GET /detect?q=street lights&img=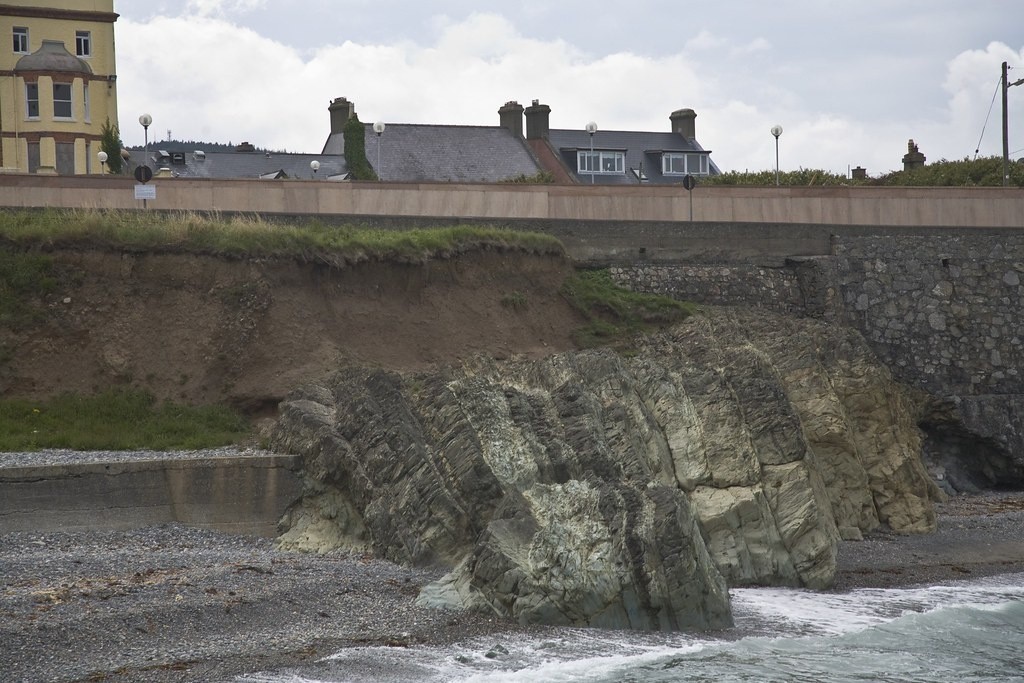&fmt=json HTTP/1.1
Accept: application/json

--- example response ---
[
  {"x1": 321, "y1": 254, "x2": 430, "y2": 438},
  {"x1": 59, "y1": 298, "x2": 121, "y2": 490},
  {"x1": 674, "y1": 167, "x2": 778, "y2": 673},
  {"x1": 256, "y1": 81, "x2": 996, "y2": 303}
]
[
  {"x1": 139, "y1": 113, "x2": 154, "y2": 167},
  {"x1": 770, "y1": 124, "x2": 784, "y2": 187},
  {"x1": 372, "y1": 120, "x2": 386, "y2": 183},
  {"x1": 309, "y1": 159, "x2": 320, "y2": 180},
  {"x1": 585, "y1": 121, "x2": 598, "y2": 186},
  {"x1": 97, "y1": 151, "x2": 109, "y2": 176}
]
